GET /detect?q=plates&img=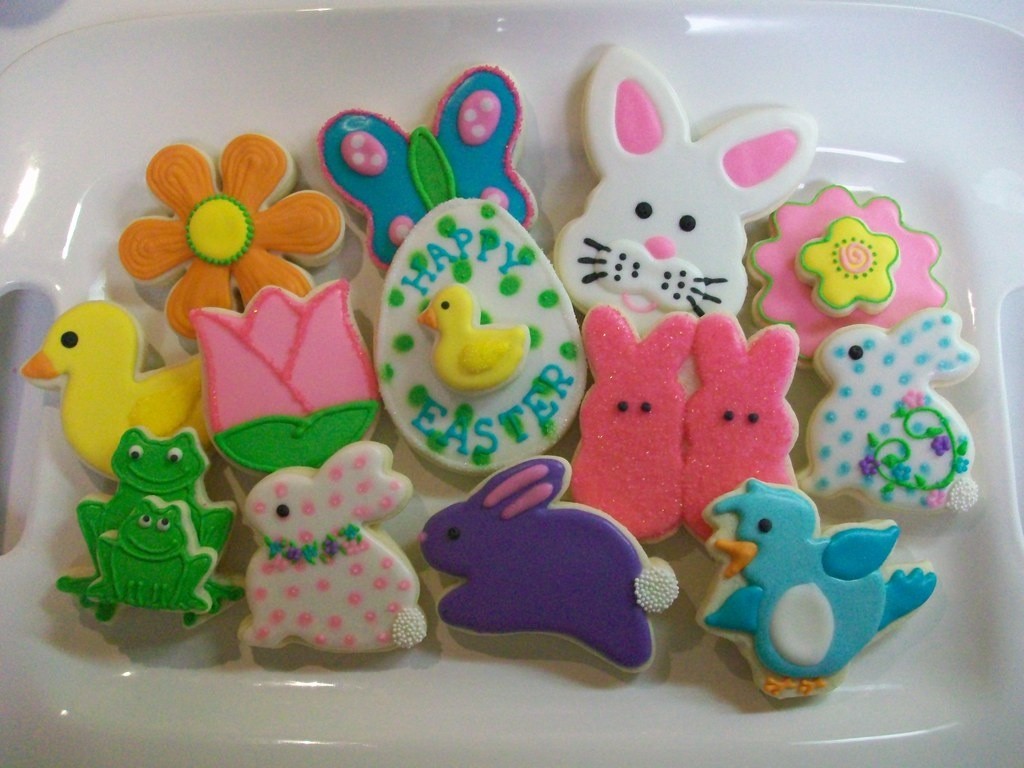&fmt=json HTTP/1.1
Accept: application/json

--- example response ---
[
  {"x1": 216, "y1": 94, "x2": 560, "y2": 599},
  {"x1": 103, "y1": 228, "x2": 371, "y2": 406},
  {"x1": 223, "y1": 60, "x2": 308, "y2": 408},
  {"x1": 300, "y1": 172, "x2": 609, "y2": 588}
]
[{"x1": 1, "y1": 2, "x2": 1024, "y2": 768}]
